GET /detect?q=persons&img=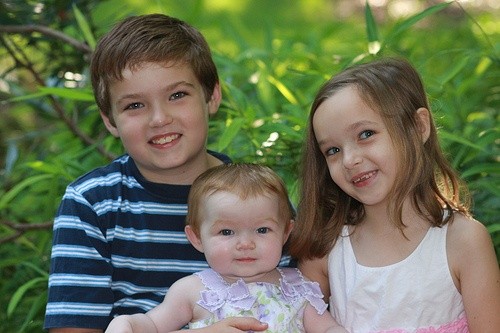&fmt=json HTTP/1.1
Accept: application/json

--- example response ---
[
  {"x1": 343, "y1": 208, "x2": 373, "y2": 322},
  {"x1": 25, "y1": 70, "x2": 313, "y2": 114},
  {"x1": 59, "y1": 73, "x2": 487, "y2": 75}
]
[
  {"x1": 287, "y1": 54, "x2": 500, "y2": 333},
  {"x1": 45, "y1": 12, "x2": 298, "y2": 333},
  {"x1": 105, "y1": 162, "x2": 351, "y2": 333}
]
